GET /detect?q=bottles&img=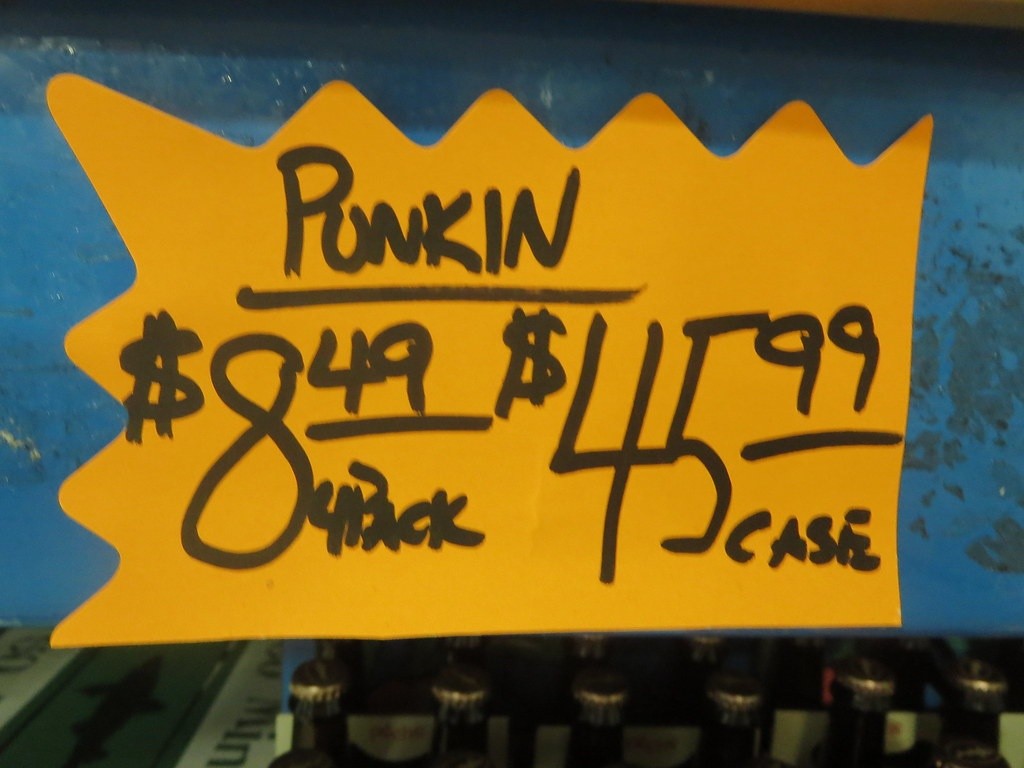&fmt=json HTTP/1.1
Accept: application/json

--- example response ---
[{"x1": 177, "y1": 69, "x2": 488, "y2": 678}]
[{"x1": 270, "y1": 637, "x2": 1022, "y2": 766}]
